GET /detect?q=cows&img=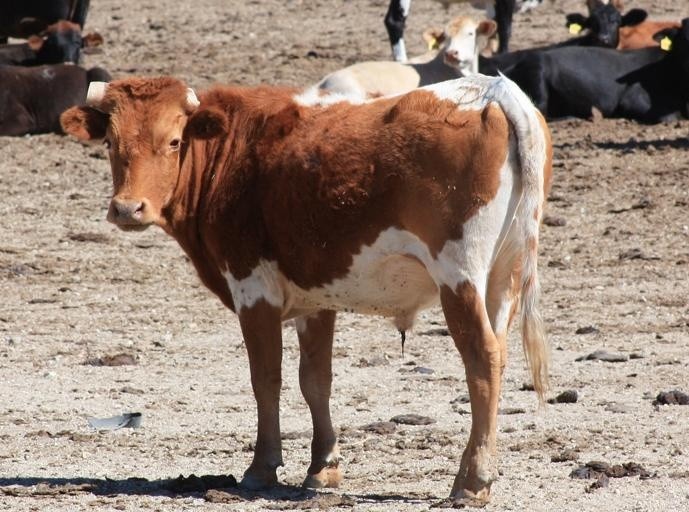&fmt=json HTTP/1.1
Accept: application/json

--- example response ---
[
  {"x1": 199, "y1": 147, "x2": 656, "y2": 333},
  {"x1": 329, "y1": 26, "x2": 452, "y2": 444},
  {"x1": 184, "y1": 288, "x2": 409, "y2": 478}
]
[
  {"x1": 479, "y1": 0, "x2": 649, "y2": 68},
  {"x1": 609, "y1": 0, "x2": 681, "y2": 49},
  {"x1": 1, "y1": 0, "x2": 115, "y2": 140},
  {"x1": 55, "y1": 66, "x2": 554, "y2": 510},
  {"x1": 505, "y1": 16, "x2": 689, "y2": 127},
  {"x1": 315, "y1": 17, "x2": 496, "y2": 103},
  {"x1": 382, "y1": 0, "x2": 544, "y2": 61}
]
